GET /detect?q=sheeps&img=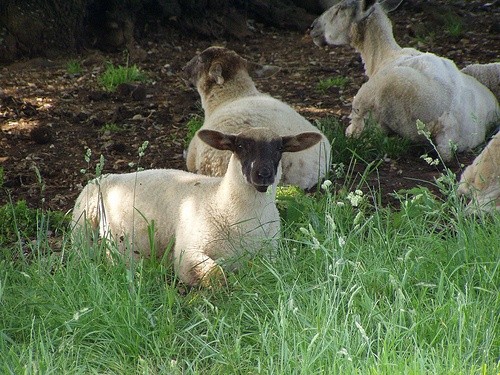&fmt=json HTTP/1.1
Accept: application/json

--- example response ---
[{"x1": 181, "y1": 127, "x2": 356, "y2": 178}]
[
  {"x1": 69, "y1": 126, "x2": 323, "y2": 289},
  {"x1": 455, "y1": 61, "x2": 500, "y2": 222},
  {"x1": 177, "y1": 44, "x2": 333, "y2": 193},
  {"x1": 309, "y1": 0, "x2": 500, "y2": 169}
]
